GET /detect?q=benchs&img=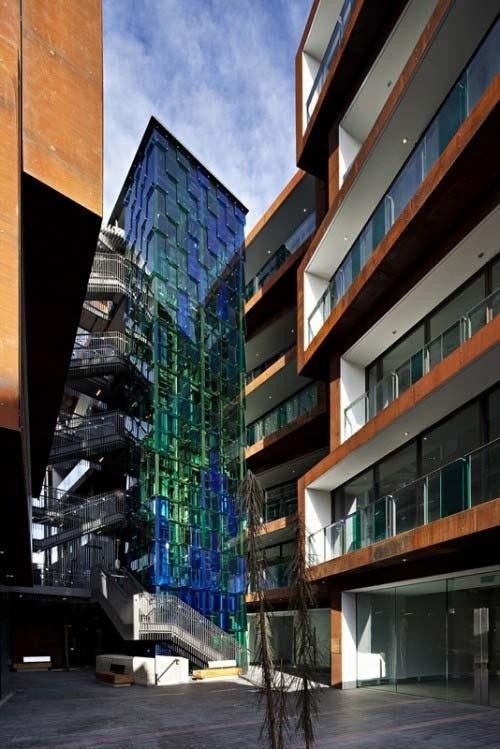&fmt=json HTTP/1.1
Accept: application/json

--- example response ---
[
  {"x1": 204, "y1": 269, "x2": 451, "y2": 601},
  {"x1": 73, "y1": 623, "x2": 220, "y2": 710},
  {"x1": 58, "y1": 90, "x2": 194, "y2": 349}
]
[
  {"x1": 192, "y1": 668, "x2": 242, "y2": 682},
  {"x1": 13, "y1": 662, "x2": 52, "y2": 672},
  {"x1": 95, "y1": 671, "x2": 135, "y2": 687}
]
